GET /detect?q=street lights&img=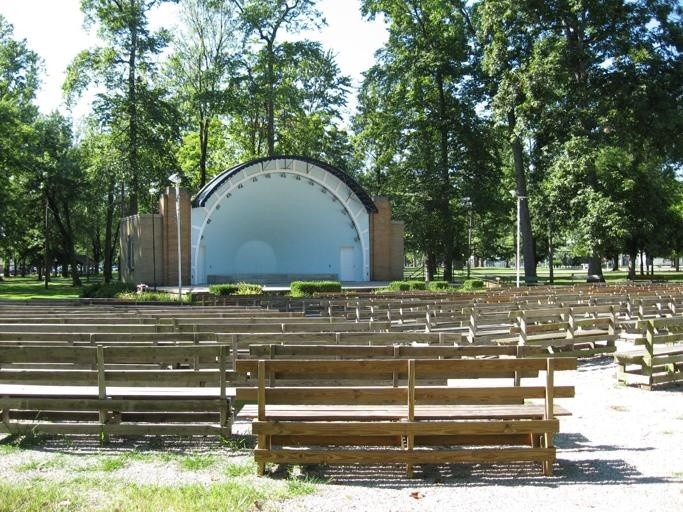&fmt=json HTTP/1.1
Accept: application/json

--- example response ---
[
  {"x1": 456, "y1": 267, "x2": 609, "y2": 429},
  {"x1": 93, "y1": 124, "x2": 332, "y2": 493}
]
[
  {"x1": 166, "y1": 171, "x2": 183, "y2": 303},
  {"x1": 508, "y1": 189, "x2": 525, "y2": 290}
]
